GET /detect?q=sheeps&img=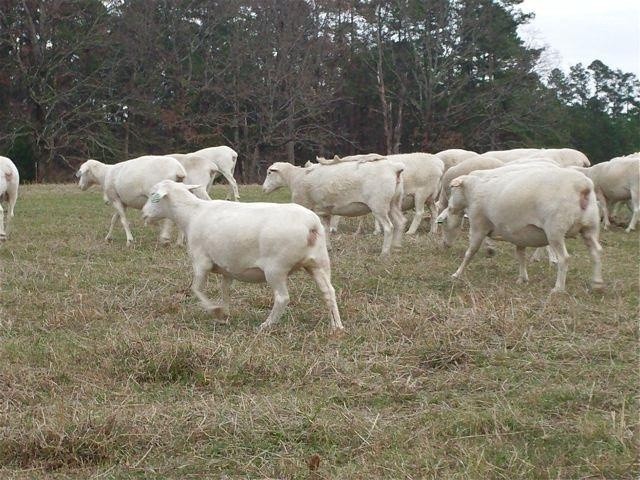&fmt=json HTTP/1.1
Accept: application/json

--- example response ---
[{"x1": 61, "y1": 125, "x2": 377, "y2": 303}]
[
  {"x1": 140, "y1": 179, "x2": 344, "y2": 333},
  {"x1": 0, "y1": 156, "x2": 19, "y2": 243},
  {"x1": 260, "y1": 148, "x2": 640, "y2": 293},
  {"x1": 75, "y1": 145, "x2": 241, "y2": 247}
]
